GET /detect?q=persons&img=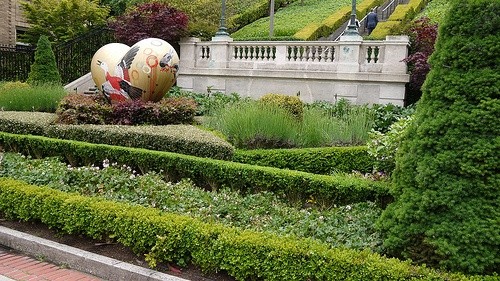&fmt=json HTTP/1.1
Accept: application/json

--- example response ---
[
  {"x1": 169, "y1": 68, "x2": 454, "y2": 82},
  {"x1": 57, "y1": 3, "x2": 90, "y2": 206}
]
[{"x1": 364, "y1": 7, "x2": 381, "y2": 37}]
